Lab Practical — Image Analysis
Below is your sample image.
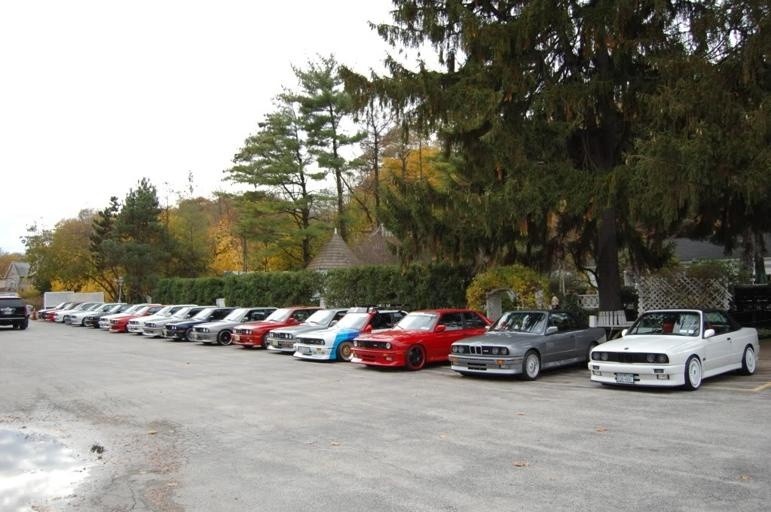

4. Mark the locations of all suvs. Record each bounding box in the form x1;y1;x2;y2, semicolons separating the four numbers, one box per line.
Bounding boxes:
1;291;30;329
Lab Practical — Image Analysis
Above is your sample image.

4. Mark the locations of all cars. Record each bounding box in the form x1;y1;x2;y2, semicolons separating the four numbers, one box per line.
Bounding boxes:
589;308;760;389
449;310;606;380
